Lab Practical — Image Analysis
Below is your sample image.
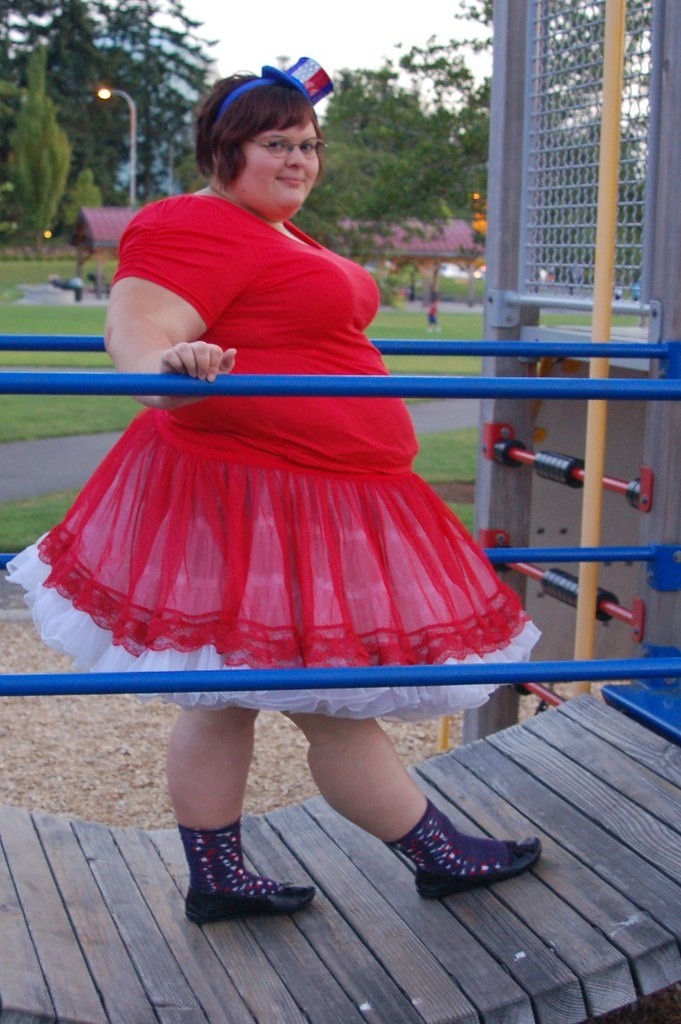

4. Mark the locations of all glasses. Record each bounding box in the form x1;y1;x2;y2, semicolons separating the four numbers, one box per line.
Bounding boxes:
248;137;327;159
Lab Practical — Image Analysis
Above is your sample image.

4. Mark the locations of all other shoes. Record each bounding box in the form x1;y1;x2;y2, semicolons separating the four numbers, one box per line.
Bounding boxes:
186;881;316;923
417;835;542;897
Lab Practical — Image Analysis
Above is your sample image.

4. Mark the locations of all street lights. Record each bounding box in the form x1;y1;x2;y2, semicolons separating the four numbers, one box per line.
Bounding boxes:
97;88;138;207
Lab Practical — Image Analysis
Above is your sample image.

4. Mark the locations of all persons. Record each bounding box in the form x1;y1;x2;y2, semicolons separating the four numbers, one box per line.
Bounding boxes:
5;57;542;923
426;297;440;332
